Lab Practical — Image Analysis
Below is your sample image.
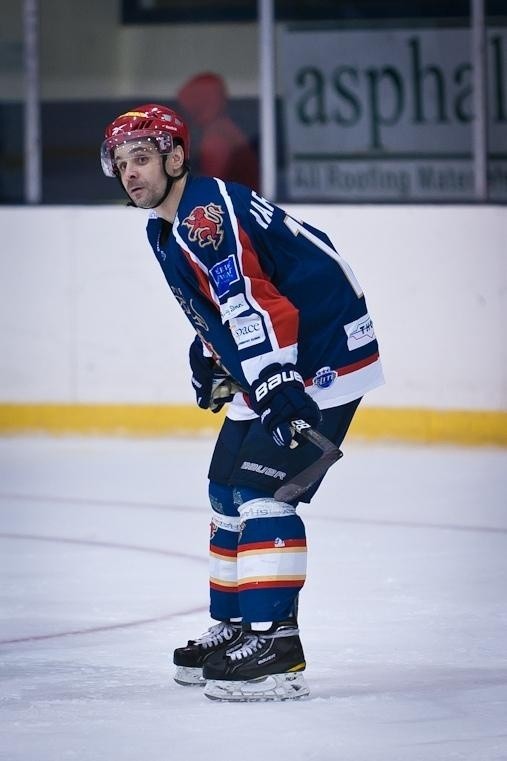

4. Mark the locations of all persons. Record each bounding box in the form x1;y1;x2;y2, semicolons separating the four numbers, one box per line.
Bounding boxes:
175;69;265;196
97;101;389;683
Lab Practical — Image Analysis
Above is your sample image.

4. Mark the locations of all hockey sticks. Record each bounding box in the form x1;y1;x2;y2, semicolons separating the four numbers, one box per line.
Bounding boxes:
225;375;343;501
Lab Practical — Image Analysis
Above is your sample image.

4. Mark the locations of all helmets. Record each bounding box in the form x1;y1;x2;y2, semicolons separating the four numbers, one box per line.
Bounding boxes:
100;103;191;178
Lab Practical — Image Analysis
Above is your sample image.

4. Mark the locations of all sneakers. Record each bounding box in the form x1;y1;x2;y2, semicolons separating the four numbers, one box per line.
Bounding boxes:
173;617;243;668
202;618;307;682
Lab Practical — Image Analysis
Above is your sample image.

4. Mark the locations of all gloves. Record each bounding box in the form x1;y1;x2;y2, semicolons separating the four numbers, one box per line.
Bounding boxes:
189;333;241;414
248;362;323;448
